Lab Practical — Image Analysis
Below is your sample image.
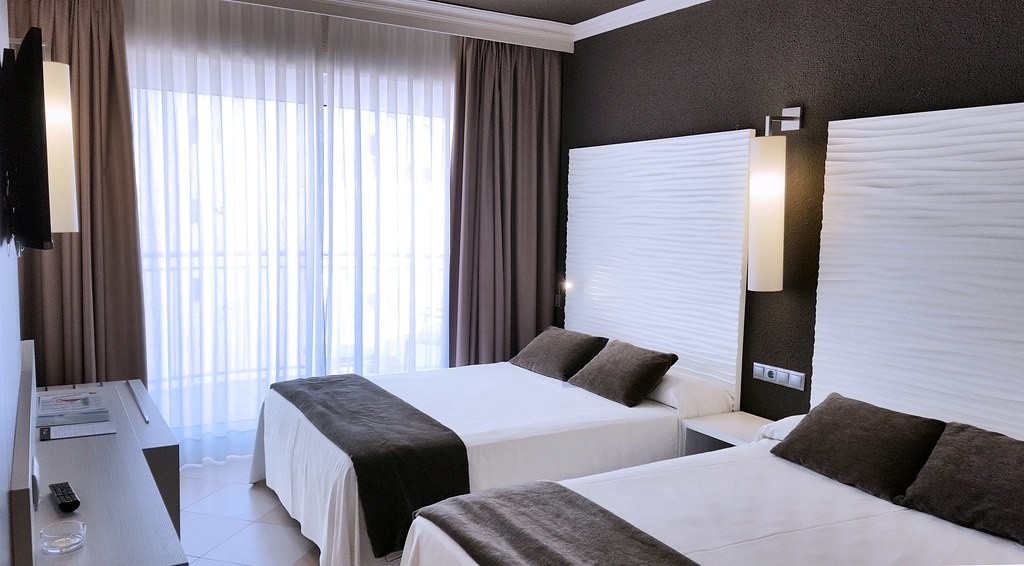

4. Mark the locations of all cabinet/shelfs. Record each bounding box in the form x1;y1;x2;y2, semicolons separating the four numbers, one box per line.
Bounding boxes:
10;339;188;566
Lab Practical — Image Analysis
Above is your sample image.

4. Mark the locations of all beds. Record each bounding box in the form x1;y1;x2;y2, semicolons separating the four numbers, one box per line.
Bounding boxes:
248;361;735;566
398;414;1024;566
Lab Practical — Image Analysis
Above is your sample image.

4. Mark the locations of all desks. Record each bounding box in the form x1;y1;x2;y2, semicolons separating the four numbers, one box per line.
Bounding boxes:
681;410;774;456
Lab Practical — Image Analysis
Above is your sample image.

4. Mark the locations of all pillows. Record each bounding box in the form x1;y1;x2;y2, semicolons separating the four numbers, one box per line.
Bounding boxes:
770;393;948;504
756;414;806;443
893;422;1024;546
650;370;732;418
568;340;679;408
509;326;609;382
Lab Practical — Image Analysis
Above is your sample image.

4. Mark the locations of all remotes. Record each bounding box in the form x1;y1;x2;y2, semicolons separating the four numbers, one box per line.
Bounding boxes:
47;481;82;513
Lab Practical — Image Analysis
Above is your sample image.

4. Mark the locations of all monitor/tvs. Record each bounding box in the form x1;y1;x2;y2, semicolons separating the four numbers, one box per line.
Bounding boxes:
13;28;55;250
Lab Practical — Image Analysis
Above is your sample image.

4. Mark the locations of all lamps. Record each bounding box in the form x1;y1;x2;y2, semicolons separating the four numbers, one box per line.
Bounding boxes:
9;37;79;232
748;107;802;292
